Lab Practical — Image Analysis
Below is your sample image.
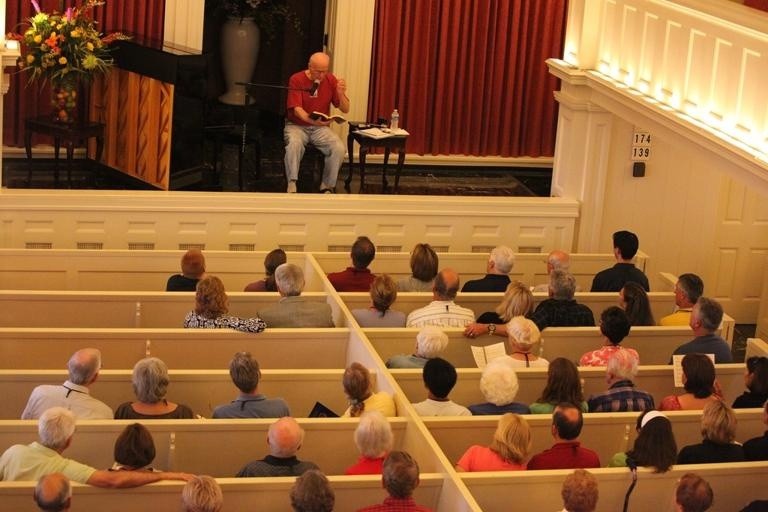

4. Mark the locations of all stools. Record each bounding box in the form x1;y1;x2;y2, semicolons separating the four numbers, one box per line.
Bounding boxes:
268;140;322;191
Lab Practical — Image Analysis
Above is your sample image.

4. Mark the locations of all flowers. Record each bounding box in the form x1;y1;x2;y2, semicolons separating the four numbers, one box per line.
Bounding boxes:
1;1;120;86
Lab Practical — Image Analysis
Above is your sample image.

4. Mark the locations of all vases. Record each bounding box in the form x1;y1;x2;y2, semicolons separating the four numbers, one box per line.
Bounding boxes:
48;83;79;123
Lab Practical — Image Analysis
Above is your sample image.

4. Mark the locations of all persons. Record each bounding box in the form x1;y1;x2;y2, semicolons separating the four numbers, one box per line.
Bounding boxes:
20;347;113;421
0;407;198;490
282;51;350;193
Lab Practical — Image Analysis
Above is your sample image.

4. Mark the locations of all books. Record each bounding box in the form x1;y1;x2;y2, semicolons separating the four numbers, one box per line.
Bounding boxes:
351;127;409;140
309;111;345;124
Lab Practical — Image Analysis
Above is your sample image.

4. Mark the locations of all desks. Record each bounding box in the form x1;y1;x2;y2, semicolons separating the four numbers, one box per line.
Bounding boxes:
18;116;107;185
344;121;406;192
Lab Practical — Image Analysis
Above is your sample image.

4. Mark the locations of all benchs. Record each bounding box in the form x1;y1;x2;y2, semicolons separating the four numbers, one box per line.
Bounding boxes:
0;244;765;510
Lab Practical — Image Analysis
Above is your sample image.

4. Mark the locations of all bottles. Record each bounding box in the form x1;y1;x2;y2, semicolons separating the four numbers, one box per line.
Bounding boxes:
391;108;400;132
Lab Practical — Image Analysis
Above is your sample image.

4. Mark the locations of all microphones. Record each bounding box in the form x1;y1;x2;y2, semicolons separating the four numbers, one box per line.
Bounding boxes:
310;79;320;95
